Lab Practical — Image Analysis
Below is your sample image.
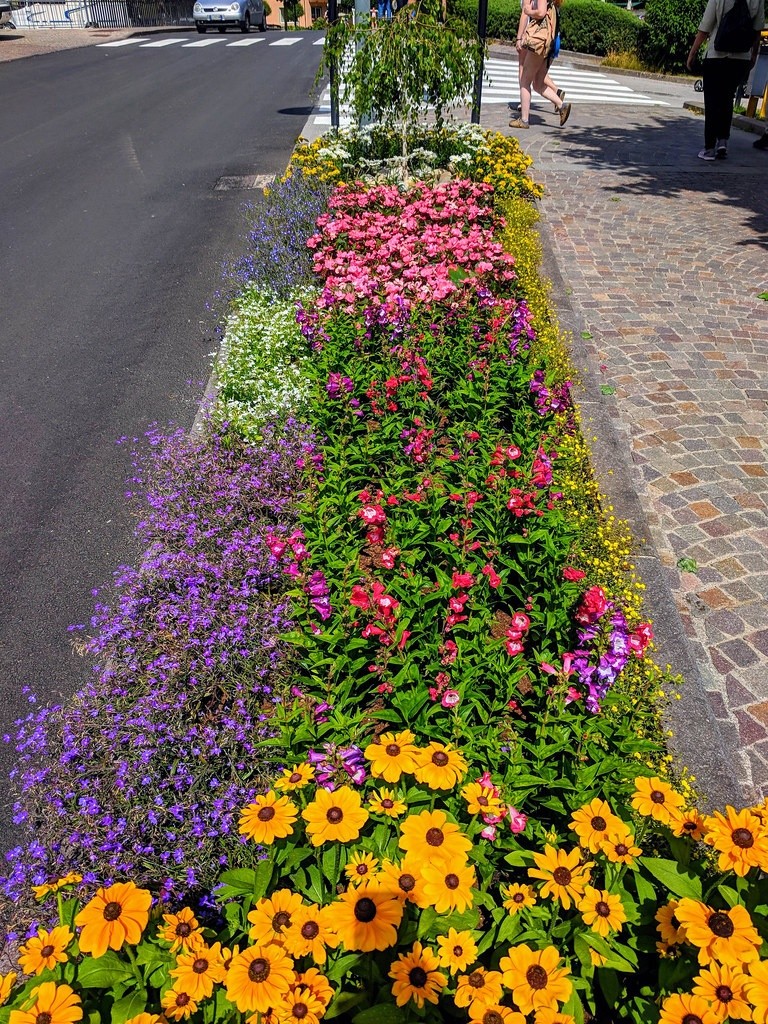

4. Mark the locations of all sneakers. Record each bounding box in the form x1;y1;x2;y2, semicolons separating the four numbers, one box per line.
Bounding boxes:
698;147;716;161
554;89;565;113
716;139;728;160
509;116;530;129
558;103;571;127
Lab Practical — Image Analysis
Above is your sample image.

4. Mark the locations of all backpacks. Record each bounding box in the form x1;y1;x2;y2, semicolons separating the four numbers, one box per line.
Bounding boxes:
714;0;757;54
519;0;556;59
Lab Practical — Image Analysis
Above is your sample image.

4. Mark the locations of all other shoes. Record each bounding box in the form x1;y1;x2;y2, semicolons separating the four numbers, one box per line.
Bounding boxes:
753;130;768;150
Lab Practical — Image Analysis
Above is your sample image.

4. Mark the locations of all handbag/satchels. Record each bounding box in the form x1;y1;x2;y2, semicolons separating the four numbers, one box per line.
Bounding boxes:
549;28;562;59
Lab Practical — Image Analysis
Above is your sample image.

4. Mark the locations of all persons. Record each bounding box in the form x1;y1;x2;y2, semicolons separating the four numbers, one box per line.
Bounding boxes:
686;0;764;160
508;0;565;112
509;0;572;129
370;7;378;29
406;0;447;101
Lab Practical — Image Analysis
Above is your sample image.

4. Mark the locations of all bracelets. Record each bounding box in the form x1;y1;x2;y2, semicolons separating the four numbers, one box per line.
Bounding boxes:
516;38;521;41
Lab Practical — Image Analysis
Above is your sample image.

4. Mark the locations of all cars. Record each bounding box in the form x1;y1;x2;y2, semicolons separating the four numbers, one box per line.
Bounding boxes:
193;0;272;35
